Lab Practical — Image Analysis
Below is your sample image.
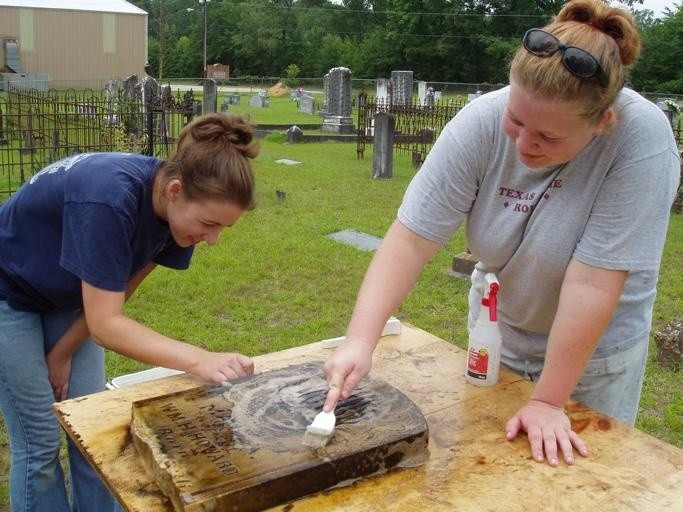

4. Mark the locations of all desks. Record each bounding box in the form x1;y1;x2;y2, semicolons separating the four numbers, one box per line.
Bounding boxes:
51;322;683;511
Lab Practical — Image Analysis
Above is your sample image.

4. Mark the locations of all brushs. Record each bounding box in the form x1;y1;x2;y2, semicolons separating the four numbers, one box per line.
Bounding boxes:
302;399;338;449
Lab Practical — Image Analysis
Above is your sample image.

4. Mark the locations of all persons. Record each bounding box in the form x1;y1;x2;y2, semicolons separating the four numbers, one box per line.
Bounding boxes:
0;111;260;512
323;1;681;467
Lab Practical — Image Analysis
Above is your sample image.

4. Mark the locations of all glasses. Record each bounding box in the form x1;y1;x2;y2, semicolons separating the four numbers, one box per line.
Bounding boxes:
524;28;608;89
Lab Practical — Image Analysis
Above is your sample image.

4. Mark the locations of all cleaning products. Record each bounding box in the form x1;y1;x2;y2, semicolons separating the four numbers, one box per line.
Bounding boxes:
465;272;502;386
